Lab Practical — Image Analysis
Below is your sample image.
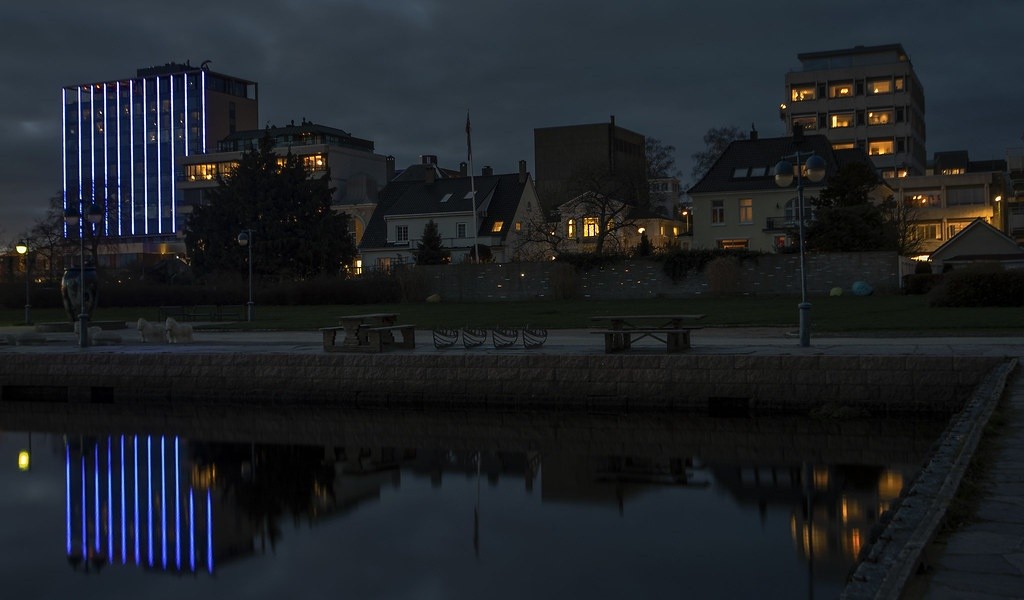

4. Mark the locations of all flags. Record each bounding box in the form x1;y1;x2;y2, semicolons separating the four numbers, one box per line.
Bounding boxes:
465;113;472;163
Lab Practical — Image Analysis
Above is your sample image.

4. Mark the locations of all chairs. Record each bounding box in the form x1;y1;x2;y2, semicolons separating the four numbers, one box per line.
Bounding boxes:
165;317;193;344
137;318;167;344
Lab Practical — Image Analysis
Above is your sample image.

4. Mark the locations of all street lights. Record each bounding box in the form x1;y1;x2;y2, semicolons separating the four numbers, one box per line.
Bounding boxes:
773;150;827;347
238;228;257;321
63;199;103;348
15;238;33;323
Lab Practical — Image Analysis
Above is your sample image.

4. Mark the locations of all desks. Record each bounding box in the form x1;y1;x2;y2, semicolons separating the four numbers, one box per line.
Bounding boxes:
590;313;706;352
340;312;401;352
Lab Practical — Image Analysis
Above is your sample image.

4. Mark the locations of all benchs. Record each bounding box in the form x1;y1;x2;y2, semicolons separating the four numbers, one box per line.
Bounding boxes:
365;324;417;352
87;325;122;345
188;305;218;321
319;324;369;352
432;325;550;350
216;304;244;322
621;326;705;347
590;330;689;352
158;306;188;323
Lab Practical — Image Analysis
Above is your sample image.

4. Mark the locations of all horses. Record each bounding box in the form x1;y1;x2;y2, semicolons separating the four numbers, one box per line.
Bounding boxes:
165;317;195;344
137;317;153;343
